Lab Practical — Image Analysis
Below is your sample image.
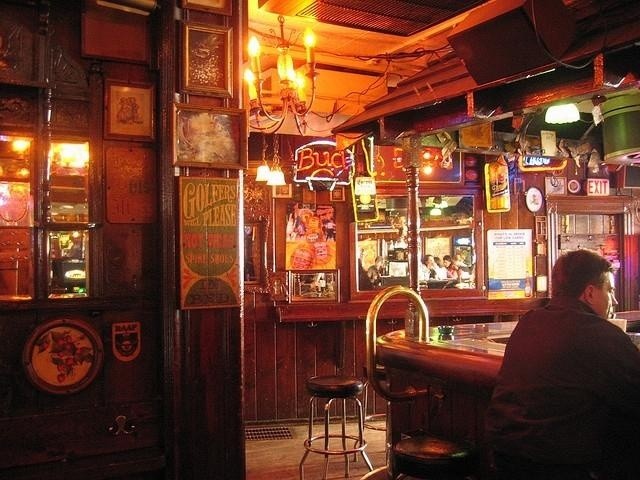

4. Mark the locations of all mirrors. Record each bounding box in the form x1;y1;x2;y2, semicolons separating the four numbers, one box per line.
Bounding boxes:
348;186;487;302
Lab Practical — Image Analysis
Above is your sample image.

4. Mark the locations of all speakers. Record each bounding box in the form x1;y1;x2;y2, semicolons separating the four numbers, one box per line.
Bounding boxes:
446;0;576;85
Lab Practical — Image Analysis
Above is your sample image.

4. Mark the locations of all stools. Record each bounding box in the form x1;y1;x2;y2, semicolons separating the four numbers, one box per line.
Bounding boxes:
391;433;478;480
299;375;374;480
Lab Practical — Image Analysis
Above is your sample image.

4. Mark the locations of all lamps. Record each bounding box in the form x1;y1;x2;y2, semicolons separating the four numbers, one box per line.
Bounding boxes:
245;13;321;188
429;194;450;221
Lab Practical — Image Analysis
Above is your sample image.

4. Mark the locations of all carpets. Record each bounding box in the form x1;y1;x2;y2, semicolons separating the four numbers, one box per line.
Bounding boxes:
243;424;297;443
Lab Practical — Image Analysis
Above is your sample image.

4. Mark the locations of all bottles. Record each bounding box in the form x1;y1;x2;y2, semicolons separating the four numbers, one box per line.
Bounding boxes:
525;271;532;297
348;139;379;224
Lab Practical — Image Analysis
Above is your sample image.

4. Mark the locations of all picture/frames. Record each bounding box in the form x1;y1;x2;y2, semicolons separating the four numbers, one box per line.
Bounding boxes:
171;103;248;170
102;78;157;144
245;216;272;294
287;270;340;304
180;21;233;100
177;176;244;311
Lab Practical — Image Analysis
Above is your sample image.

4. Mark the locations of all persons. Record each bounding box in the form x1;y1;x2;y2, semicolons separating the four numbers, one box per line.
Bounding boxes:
418;254;461;287
367;256;385;288
326;220;336;242
486;248;640;480
